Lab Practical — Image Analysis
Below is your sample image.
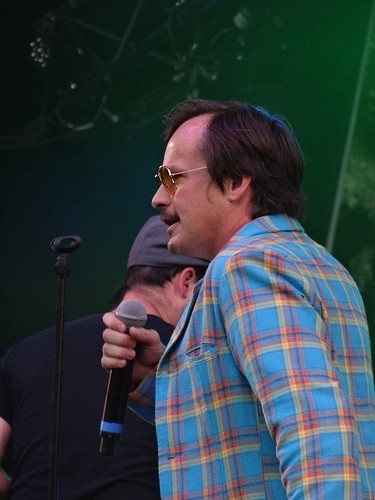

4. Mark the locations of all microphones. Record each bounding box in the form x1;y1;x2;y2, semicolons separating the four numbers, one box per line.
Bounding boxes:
97;300;148;471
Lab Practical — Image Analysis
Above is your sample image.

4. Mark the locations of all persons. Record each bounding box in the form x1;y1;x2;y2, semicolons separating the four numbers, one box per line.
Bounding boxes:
0;214;212;500
100;98;375;500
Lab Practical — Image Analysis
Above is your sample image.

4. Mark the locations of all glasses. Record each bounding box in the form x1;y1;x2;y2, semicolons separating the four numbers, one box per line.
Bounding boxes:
155;165;211;196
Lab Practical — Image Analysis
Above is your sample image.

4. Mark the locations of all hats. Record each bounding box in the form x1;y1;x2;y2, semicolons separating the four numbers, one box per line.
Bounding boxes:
127;215;211;270
